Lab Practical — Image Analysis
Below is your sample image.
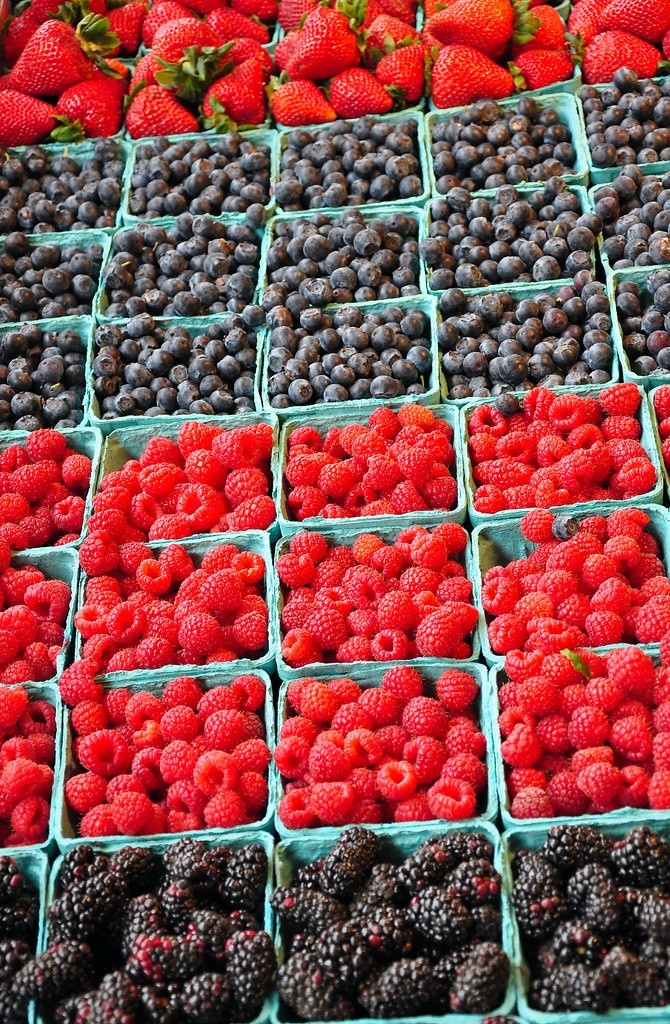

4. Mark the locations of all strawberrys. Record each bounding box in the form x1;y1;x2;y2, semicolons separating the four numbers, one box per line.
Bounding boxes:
0;0;670;150
